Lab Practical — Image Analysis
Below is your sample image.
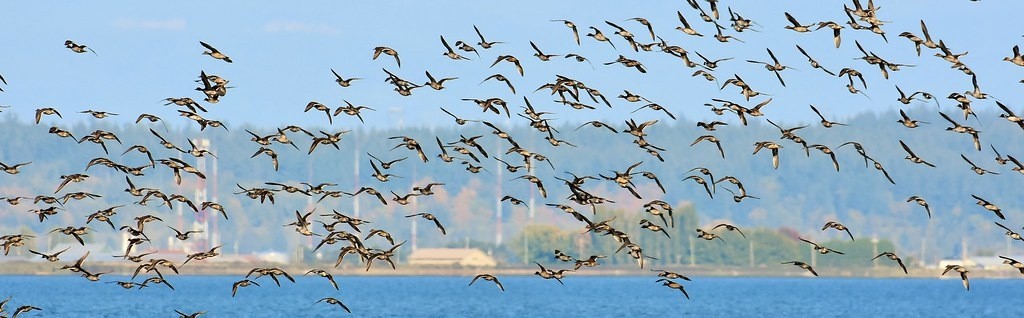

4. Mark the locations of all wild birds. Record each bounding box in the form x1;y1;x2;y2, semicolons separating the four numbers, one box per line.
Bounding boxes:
0;0;1024;318
